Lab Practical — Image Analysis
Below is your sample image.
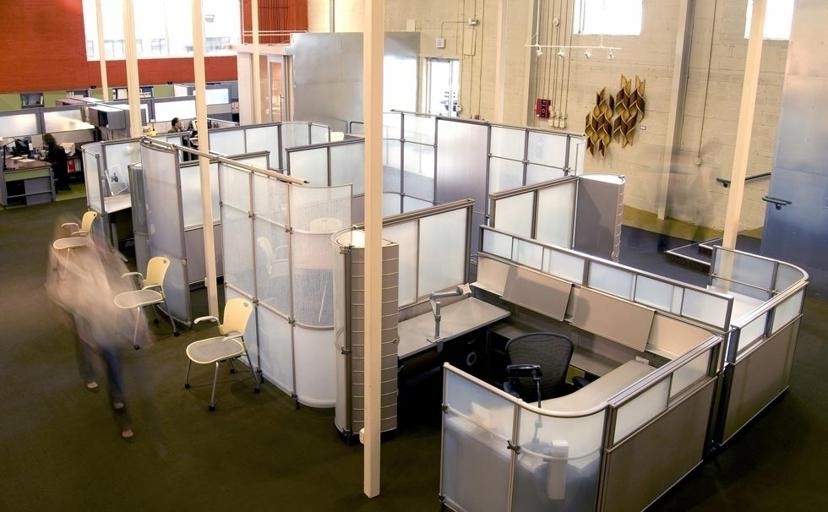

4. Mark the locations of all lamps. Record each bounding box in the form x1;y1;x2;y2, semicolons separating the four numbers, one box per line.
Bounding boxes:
506;364;550;453
522;33;624;62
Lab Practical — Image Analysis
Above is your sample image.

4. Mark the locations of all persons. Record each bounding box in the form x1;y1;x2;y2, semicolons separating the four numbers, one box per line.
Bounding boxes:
45;211;152;444
167;117;185;133
34;133;67;193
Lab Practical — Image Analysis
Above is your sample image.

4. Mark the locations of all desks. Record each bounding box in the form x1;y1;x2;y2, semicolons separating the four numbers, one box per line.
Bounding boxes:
445;359;670;475
6;149;80;208
397;296;512;360
104;192;132;247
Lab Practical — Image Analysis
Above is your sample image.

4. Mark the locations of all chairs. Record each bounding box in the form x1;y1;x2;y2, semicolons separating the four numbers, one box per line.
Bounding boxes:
258;217;342;280
503;330;592;399
184;298;259;408
115;257;179;350
54;211;102;277
